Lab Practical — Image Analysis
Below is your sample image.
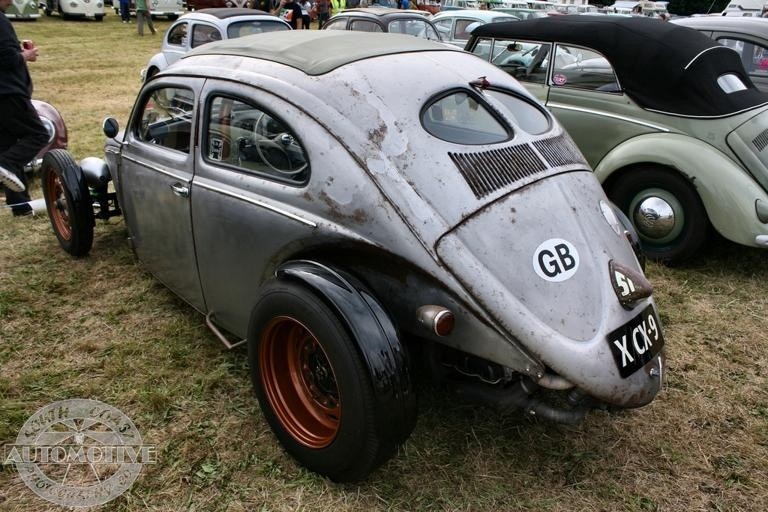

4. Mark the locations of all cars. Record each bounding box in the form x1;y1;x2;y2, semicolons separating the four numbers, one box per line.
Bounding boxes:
2;2;41;22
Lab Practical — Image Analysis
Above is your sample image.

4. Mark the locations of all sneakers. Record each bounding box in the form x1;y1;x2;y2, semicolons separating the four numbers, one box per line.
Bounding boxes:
0;164;26;193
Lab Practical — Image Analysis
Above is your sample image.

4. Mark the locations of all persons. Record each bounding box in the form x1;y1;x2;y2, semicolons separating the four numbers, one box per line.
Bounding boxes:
0;1;53;218
135;1;159;37
120;1;133;24
251;1;428;30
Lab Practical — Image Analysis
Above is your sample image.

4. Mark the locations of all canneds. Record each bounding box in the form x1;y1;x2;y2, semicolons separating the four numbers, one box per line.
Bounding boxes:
23;40;33;50
